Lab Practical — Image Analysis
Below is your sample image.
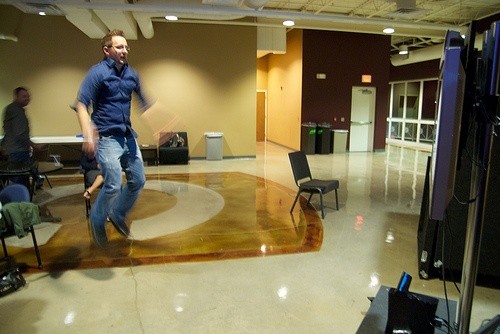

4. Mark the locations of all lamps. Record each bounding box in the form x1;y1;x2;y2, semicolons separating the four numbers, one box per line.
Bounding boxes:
399;44;409;55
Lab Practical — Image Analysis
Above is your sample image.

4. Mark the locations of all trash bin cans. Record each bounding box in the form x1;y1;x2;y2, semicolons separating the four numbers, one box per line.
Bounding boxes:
204;132;223;161
331;129;349;153
318;124;331;153
302;123;317;154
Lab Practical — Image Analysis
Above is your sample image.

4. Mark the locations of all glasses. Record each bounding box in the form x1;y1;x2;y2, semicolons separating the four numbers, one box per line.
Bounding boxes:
107;45;130;52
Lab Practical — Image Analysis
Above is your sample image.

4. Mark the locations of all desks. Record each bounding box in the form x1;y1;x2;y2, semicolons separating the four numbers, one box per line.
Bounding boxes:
356;286;458;334
31;135;85;162
330;130;348;151
140;144;158;166
35;160;64;188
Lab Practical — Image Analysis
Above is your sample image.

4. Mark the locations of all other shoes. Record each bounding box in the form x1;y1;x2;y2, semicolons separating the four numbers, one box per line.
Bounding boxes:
91;221;107;247
107;214;130;237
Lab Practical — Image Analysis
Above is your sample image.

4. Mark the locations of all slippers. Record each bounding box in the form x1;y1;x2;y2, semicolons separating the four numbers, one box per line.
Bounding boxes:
85;191;91;199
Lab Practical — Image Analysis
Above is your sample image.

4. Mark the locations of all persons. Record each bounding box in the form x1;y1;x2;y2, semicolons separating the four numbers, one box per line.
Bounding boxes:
68;28;175;248
0;86;42;236
81;112;123;223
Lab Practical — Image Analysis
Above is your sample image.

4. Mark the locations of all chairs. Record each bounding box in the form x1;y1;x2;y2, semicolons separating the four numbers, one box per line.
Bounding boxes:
0;184;42;269
81;152;123;217
288;151;340;219
159;131;189;164
